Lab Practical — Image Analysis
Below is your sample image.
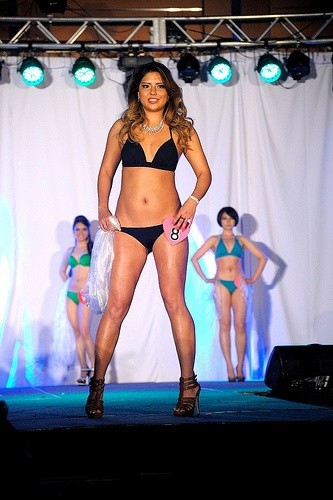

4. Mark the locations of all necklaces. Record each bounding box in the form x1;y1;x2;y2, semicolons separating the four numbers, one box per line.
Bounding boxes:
141;120;164;136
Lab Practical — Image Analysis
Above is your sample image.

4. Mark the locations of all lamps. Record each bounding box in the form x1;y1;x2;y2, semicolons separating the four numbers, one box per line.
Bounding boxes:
168;40;201;83
254;40;287;83
17;43;45;86
118;41;154;92
207;41;232;84
69;44;97;86
283;39;311;81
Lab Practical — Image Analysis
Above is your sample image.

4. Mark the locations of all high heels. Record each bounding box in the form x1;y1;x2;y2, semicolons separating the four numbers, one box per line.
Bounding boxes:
228;365;236;382
88;368;94;384
75;366;91;385
236;366;245;382
174;371;202;416
85;376;105;419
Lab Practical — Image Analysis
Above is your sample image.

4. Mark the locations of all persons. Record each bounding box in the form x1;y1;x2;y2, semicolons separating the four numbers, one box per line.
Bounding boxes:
84;62;211;418
192;207;268;382
59;216;95;383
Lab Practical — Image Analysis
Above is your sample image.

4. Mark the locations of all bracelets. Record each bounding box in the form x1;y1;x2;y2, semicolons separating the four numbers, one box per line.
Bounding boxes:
189;195;199;204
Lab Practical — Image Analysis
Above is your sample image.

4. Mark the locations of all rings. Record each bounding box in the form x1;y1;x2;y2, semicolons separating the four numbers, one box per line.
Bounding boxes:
180;216;184;220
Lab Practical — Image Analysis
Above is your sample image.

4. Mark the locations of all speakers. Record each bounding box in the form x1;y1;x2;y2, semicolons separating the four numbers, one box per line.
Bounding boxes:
264;344;333;395
